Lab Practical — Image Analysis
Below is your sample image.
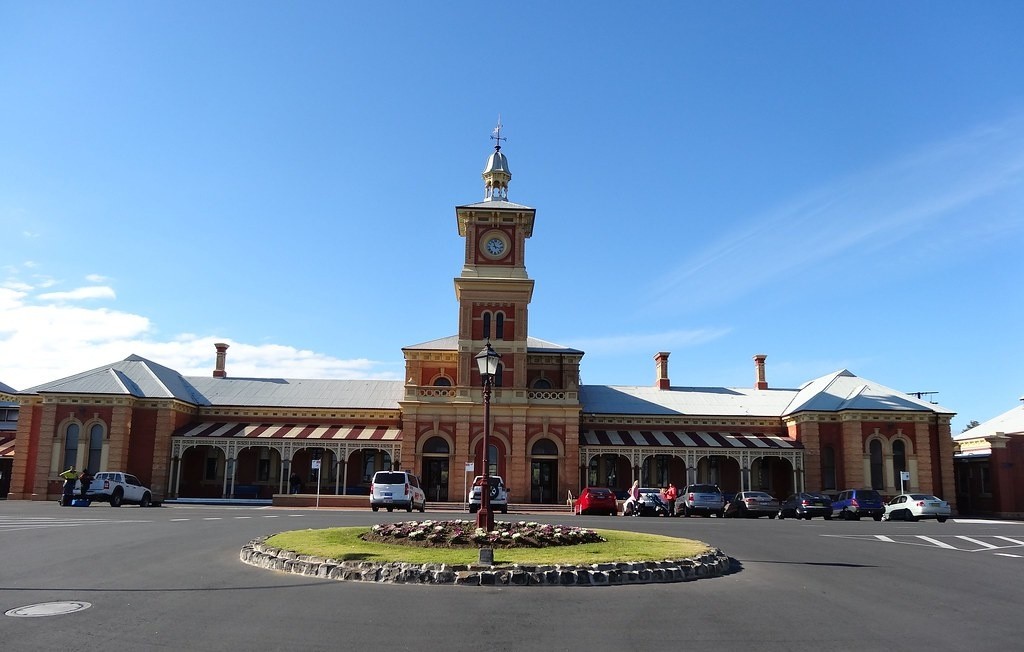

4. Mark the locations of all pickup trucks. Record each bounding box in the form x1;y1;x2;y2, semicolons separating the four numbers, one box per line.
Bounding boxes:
63;472;152;508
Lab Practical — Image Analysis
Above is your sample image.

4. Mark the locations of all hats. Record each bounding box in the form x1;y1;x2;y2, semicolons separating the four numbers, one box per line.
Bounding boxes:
83;468;88;473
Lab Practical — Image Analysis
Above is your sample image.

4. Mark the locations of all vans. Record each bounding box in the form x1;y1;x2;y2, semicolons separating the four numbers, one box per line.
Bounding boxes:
369;471;426;513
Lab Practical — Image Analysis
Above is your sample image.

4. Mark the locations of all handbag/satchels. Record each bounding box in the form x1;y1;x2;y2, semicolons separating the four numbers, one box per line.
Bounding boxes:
71;499;92;507
627;488;642;498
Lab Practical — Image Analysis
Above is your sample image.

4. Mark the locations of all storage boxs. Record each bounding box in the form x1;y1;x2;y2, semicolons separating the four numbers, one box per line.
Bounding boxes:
72;499;89;507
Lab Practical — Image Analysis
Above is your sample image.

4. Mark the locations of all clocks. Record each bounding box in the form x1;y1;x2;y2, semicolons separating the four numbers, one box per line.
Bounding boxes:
487;238;504;255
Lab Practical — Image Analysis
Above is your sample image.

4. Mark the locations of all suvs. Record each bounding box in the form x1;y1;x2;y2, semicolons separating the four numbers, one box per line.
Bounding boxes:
469;476;510;514
673;484;725;518
831;489;886;522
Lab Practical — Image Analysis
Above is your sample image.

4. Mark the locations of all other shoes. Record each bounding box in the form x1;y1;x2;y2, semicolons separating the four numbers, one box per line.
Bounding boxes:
668;513;674;516
632;513;639;516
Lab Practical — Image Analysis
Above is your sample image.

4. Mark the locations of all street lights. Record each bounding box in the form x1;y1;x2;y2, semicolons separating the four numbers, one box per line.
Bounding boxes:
474;337;502;532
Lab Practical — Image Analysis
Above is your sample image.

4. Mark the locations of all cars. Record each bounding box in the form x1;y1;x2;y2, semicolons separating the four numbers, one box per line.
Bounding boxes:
574;487;619;516
778;492;833;520
623;488;668;517
882;493;951;522
733;491;780;519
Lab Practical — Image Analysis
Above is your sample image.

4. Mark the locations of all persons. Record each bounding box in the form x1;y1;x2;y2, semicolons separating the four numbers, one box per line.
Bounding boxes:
665;483;677;517
631;479;642;516
58;465;79;505
80;469;94;501
